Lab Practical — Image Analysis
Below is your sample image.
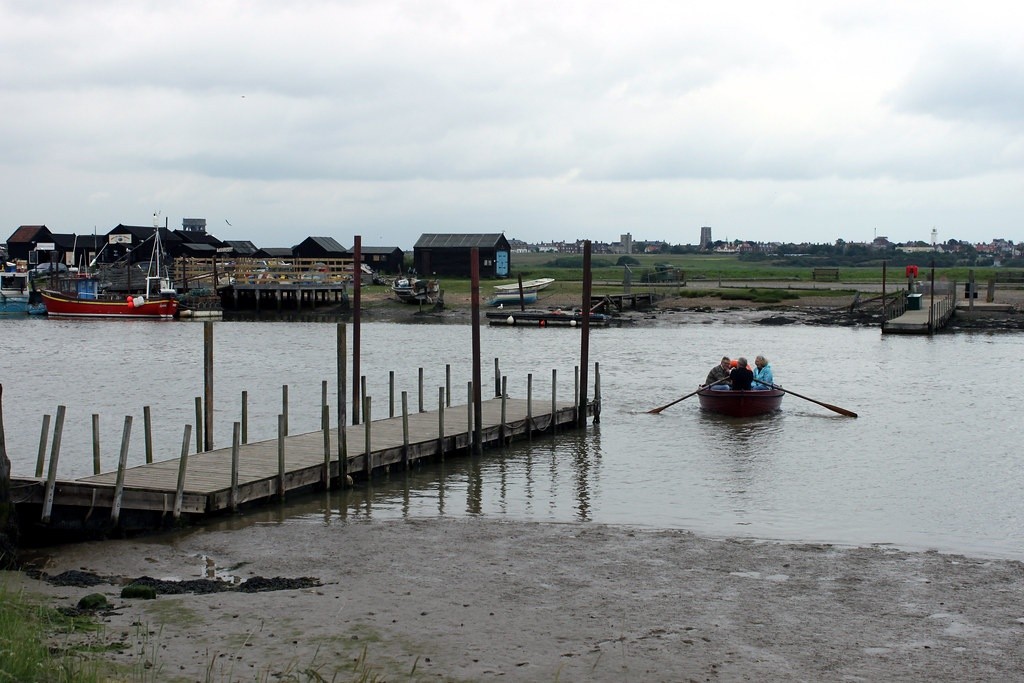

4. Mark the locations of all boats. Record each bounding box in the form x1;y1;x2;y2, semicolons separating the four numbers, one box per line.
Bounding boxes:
390;272;439;303
39;209;180;319
493;277;554;293
185;306;224;317
0;268;30;313
485;287;538;307
697;383;786;417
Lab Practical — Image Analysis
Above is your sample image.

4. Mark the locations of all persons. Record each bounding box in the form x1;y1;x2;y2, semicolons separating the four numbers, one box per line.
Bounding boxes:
730;357;754;390
706;356;732;390
751;356;773;390
907;268;914;290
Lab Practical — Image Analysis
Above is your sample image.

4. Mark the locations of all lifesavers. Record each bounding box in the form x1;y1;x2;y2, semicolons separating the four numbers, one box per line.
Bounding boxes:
76;273;90;276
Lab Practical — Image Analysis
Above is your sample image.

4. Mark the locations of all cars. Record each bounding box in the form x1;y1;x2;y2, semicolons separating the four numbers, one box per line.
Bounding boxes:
28;261;70;279
251;261;269;273
131;260;156;274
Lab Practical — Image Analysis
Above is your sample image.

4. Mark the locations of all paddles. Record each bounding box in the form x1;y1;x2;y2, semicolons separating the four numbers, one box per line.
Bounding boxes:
649;375;732;413
752;377;858;418
